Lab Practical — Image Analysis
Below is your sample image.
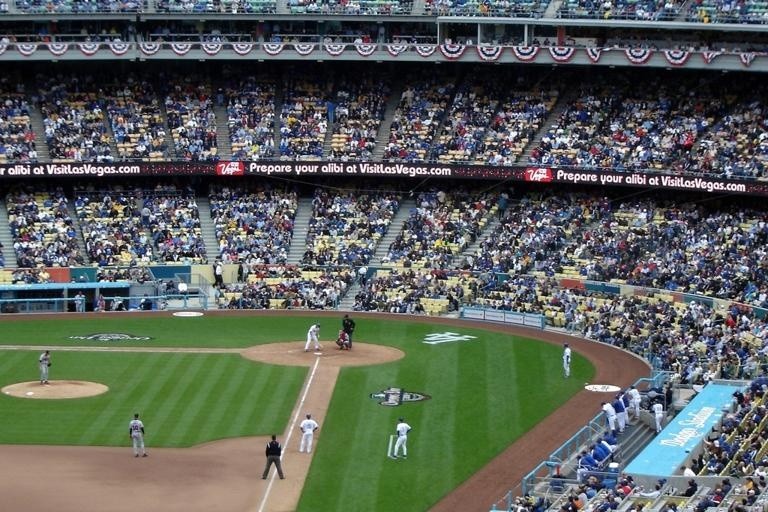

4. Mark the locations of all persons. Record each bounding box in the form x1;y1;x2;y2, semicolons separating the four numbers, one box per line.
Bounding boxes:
304;323;322;352
298;413;319;454
342;313;355;348
37;350;52;385
261;434;285;479
128;413;149;457
336;329;350;350
391;418;412;459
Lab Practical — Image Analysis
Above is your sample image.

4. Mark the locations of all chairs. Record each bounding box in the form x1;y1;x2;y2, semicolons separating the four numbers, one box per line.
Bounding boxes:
229;191;547;315
546;192;767;379
694;389;768;476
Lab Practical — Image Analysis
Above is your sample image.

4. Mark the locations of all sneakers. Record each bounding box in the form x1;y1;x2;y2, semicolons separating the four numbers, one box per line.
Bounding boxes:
136;453;148;456
392;455;408;460
40;380;49;384
